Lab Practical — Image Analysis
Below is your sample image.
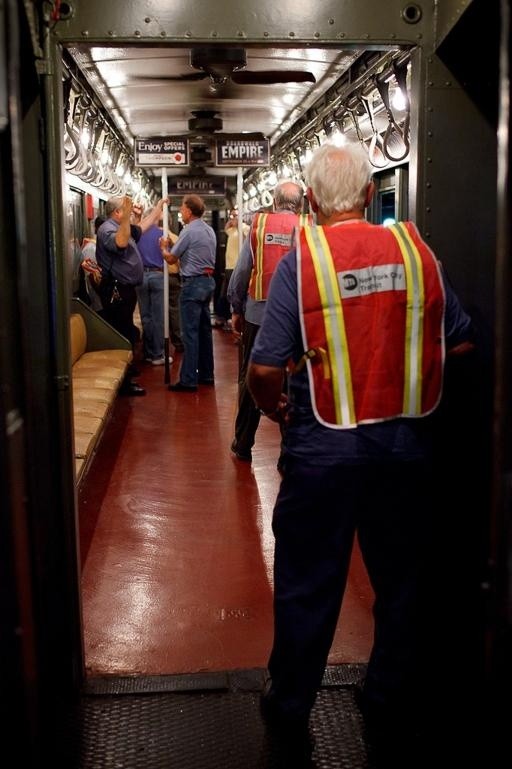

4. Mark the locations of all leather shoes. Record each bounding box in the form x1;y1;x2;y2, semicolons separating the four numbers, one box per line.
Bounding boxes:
127;381;146;399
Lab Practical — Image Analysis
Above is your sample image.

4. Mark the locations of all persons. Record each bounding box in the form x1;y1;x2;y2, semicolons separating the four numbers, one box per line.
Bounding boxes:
226;180;303;464
81;195;248;398
242;142;479;743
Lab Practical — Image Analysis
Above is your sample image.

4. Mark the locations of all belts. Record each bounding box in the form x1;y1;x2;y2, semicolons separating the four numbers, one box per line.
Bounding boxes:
145;268;163;271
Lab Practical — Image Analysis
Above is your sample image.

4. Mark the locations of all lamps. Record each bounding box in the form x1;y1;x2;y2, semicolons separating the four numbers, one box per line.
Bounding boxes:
390;77;406;111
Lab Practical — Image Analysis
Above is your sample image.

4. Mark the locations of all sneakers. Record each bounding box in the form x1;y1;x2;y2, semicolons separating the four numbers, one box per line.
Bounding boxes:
355;678;367;693
261;678;275;697
169;377;214;391
231;439;251;460
145;357;174;365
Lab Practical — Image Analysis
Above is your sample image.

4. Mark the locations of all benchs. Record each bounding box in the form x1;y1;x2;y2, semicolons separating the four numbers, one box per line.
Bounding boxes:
69;313;133;576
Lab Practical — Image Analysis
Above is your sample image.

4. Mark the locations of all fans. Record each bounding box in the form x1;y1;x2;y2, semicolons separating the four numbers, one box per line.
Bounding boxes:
127;46;316;92
147;111;266;142
